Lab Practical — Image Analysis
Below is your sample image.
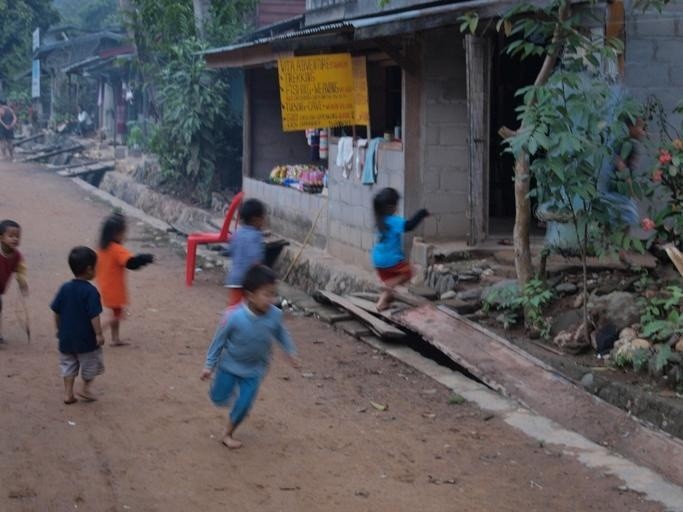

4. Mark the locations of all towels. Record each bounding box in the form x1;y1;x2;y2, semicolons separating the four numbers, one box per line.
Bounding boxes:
335;136;354;180
362;136;384;186
356;138;366;179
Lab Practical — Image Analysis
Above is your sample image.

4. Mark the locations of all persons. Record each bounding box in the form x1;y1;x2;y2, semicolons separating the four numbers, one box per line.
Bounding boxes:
199;264;302;448
0;96;32;161
49;245;104;404
74;104;94;139
223;198;267;307
596;111;647;264
370;187;429;312
93;213;153;346
0;219;30;344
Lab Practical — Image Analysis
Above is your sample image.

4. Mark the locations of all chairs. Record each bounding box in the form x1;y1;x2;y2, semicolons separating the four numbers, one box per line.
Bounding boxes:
185;191;244;287
